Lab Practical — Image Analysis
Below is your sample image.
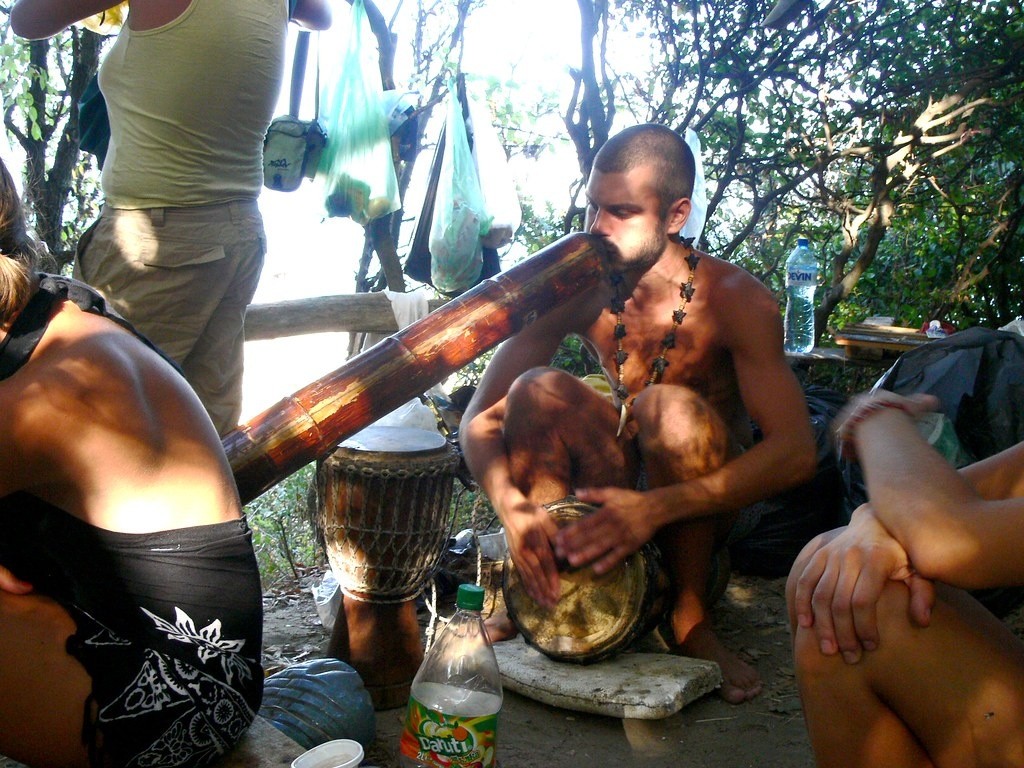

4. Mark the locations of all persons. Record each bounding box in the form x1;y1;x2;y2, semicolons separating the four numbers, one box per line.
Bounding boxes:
12;1;330;445
785;391;1023;768
460;125;815;703
0;158;263;768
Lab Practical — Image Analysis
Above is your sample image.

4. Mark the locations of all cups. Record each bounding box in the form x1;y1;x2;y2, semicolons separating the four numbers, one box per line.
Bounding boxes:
291;739;364;768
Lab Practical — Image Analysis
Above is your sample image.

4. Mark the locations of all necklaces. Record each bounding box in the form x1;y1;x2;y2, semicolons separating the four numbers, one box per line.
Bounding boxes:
608;235;703;439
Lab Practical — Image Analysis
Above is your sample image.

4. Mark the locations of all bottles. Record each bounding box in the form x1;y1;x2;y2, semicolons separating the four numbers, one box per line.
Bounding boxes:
400;584;504;768
782;239;817;354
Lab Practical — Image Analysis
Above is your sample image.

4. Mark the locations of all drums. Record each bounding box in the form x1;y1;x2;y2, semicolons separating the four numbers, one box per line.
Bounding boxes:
490;494;676;663
316;425;459;710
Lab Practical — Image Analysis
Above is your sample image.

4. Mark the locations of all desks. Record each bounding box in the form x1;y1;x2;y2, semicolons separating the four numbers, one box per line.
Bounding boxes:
786;345;894;383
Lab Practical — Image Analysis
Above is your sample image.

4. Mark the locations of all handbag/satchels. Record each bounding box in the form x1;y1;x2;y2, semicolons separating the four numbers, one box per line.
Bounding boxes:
77;69;110;171
262;115;328;192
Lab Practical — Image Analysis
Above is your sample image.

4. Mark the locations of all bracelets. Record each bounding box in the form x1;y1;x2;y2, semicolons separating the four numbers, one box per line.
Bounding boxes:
836;399;916;463
